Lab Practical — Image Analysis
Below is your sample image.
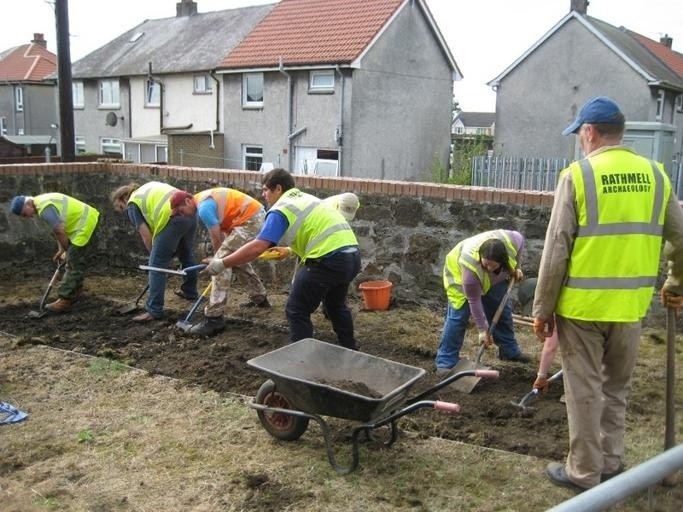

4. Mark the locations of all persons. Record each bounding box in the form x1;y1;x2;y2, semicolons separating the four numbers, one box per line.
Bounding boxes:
526;96;683;497
195;167;362;352
289;193;361;321
108;182;200;323
8;191;102;315
427;227;534;381
515;278;560;398
166;185;274;340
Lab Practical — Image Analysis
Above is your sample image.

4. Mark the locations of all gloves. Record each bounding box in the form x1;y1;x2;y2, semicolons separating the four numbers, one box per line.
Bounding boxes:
532;373;549;402
533;316;555;343
198;257;226;276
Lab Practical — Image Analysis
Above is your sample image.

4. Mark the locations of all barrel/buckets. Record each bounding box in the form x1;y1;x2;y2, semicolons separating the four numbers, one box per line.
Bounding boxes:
358;279;392;312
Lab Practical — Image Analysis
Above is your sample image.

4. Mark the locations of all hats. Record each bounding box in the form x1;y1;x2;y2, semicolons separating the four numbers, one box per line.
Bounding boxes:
169;191;187;217
561;97;625;136
338;193;360;220
11;196;25;216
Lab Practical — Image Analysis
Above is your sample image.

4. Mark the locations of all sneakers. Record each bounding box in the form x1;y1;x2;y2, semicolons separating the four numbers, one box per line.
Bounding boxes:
512;352;529;363
44;298;72;313
239;296;270;309
190;316;226;339
437;368;451;378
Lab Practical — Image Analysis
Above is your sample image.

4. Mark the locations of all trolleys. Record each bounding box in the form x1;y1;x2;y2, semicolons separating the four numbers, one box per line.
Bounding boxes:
244;335;501;476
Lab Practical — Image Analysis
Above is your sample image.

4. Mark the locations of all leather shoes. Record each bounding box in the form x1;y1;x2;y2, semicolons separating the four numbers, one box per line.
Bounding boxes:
132;313;159;322
601;463;624;482
545;461;585;494
175;290;195;300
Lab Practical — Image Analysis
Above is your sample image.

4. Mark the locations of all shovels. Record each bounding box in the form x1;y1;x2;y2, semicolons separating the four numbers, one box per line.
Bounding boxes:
441;271;515;393
174;279;212;331
27;257;66;318
117;282;150;314
138;247;281;276
